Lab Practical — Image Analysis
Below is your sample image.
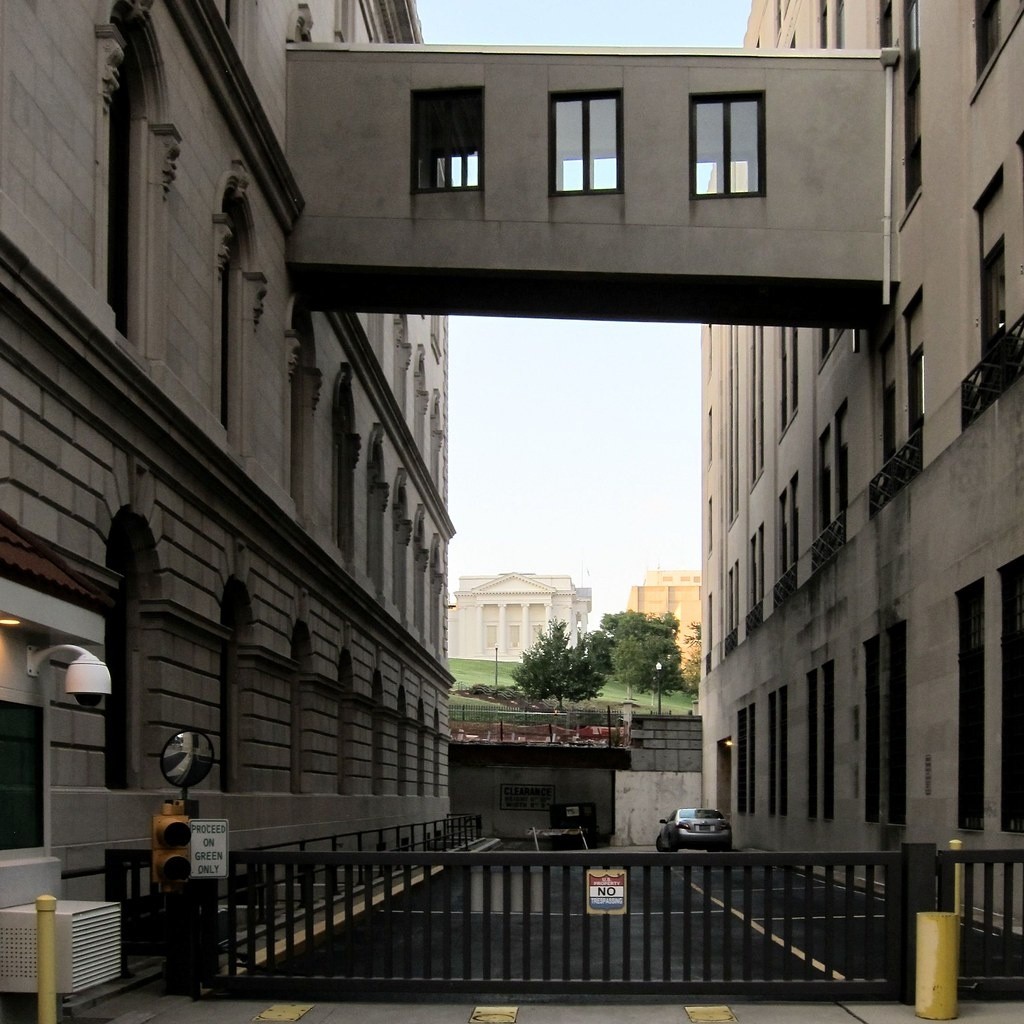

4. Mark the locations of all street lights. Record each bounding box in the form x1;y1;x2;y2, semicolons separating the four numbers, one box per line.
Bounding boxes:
655;662;662;715
494;643;499;685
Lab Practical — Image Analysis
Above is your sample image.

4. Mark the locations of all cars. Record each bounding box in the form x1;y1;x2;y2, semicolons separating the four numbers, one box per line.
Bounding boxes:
655;808;733;852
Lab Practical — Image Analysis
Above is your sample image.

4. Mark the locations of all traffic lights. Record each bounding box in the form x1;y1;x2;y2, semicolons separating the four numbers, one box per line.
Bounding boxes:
150;798;193;894
